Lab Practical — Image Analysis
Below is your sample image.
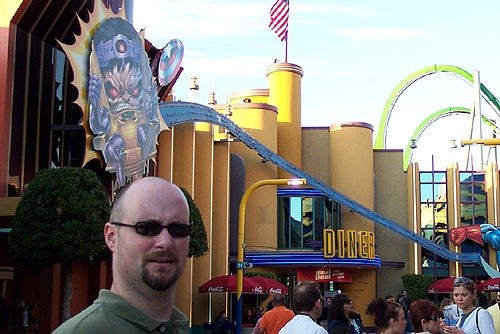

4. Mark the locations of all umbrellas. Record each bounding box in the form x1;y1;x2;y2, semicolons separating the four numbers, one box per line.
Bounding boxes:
429;277;500;293
198;273;288;294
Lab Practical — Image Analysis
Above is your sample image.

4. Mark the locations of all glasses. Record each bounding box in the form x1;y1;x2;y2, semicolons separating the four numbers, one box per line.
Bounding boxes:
454;278;475;291
425;313;441;321
342;299;351;304
110;221;193;237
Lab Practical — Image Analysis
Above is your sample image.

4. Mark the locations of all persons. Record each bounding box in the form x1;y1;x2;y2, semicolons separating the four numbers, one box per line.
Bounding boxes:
410;299;443;334
50;176;193;334
326;290;369;334
442;276;496;334
385;288;500;334
278;280;329;334
365;298;407;334
210;310;228;334
243;293;295;334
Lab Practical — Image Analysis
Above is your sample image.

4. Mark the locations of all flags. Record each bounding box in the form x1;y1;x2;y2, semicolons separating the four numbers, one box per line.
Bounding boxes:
269;0;290;42
